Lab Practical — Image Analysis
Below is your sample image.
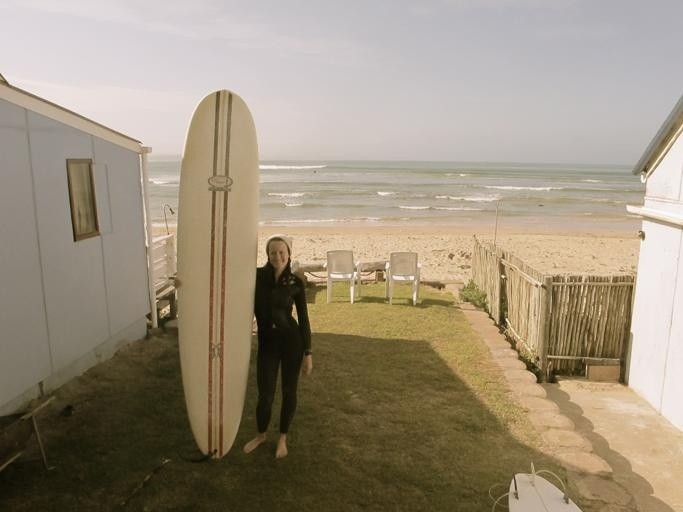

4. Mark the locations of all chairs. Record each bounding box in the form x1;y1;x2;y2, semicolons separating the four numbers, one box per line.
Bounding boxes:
325;250;422;306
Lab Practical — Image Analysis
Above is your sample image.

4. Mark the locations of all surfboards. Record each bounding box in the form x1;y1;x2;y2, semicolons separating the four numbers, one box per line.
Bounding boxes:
177;90;260;460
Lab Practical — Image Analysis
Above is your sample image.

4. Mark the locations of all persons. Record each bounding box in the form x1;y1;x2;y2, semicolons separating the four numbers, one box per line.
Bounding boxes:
174;234;314;458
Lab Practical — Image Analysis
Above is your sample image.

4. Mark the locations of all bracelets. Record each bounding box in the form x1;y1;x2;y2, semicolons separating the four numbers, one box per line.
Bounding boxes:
305;351;313;356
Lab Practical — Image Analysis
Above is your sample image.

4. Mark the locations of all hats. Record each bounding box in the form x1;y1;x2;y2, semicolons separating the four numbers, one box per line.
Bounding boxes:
266;234;293;257
